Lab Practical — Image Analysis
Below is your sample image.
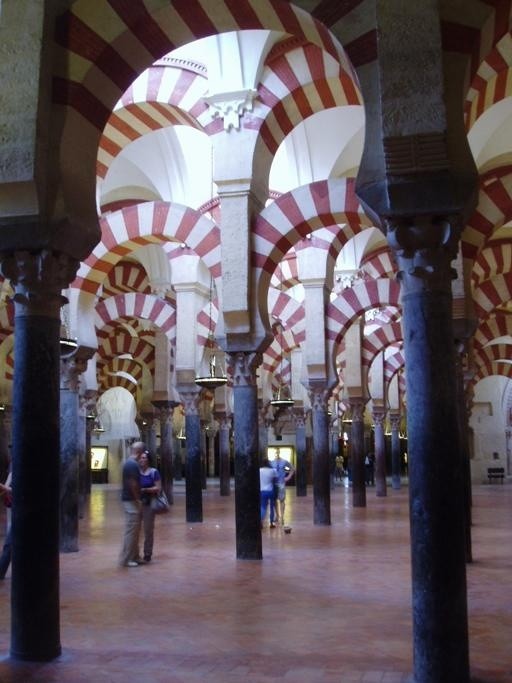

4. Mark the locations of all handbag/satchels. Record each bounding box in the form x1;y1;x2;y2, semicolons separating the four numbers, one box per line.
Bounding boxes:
151;493;170;514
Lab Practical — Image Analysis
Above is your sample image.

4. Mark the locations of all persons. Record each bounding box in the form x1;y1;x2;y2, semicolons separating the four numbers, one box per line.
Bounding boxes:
0;472;12;580
334;451;375;487
490;452;505;488
259;445;295;529
120;442;161;567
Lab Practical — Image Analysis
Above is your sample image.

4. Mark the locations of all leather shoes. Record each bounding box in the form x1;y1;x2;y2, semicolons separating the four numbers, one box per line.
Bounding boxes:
119;560;147;566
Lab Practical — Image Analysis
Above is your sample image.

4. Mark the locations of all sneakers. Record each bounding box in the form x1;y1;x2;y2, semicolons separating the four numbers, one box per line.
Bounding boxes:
144;553;151;560
268;515;284;528
